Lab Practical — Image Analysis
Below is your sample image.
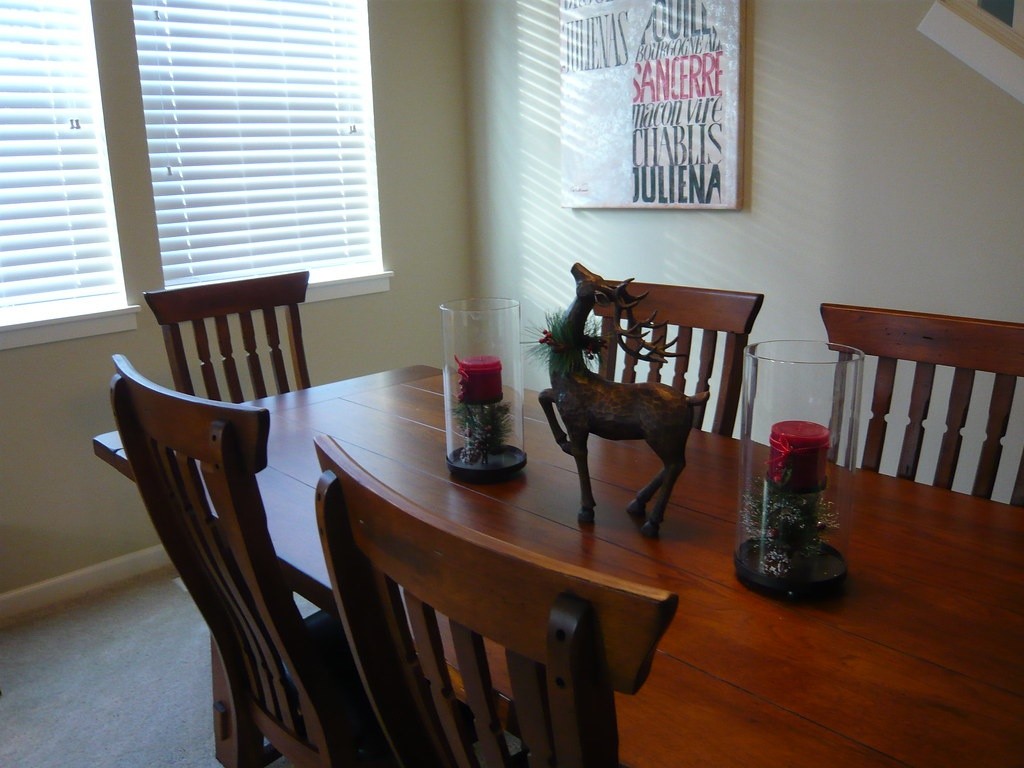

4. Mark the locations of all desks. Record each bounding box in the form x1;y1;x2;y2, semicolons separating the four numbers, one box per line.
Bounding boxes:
87;363;1024;768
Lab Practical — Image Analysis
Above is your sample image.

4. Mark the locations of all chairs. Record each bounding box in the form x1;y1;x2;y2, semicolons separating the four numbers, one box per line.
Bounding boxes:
142;270;310;406
109;353;479;768
593;280;765;437
819;303;1024;506
313;434;679;768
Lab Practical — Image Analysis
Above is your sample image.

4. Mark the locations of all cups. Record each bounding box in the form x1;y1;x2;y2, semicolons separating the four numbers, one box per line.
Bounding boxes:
733;340;868;606
436;297;527;486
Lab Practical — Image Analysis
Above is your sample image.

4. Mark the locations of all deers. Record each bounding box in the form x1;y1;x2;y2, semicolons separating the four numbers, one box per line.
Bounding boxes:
537;262;712;539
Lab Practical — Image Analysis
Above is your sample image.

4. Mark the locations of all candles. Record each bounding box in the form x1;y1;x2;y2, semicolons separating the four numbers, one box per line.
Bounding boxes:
770;421;832;496
458;356;504;407
